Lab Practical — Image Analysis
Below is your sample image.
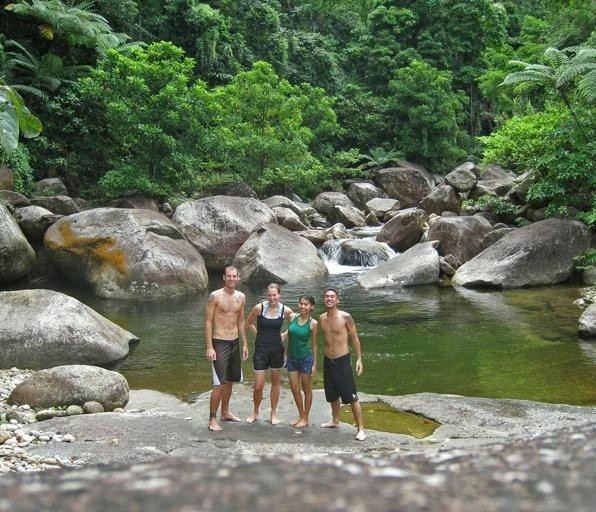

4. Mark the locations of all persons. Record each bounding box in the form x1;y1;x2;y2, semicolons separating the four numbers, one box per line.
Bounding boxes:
317;288;366;440
205;267;249;432
284;296;317;428
246;282;294;425
